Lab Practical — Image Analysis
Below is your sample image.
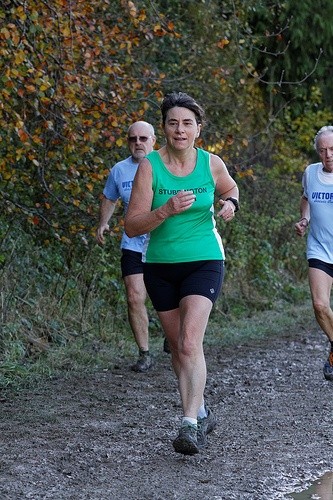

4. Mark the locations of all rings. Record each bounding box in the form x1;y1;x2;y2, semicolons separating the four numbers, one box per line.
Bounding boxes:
227;214;229;218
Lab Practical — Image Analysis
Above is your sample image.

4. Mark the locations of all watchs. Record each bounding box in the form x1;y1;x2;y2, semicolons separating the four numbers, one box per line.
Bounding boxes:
225;197;239;212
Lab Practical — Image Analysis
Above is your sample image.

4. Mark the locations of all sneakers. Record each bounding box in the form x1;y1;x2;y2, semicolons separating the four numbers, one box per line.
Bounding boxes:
197;407;217;441
173;420;199;456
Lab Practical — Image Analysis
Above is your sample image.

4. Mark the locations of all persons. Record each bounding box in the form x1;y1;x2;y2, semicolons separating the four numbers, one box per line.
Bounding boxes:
295;128;333;379
124;94;239;456
98;121;157;370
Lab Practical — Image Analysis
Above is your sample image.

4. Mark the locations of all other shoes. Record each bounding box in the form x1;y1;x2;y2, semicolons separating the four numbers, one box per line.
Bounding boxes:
132;353;153;371
163;338;171;354
323;351;333;379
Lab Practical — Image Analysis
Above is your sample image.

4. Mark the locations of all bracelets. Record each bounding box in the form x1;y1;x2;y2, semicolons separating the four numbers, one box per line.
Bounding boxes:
300;218;309;226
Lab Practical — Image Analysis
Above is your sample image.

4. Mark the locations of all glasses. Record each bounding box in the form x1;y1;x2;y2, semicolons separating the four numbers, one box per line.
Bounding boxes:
128;136;152;142
